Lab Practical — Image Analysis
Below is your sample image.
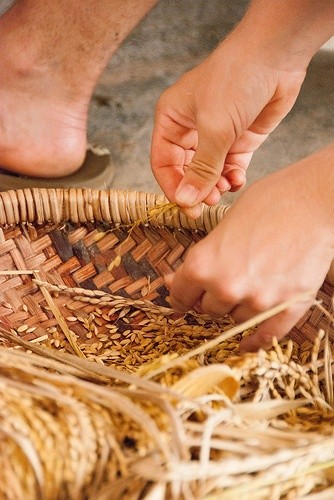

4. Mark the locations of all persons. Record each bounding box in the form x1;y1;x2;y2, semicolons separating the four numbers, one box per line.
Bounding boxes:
1;0;334;352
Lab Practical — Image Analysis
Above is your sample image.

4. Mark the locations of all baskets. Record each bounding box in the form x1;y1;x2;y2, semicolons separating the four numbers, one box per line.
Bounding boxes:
1;189;332;401
